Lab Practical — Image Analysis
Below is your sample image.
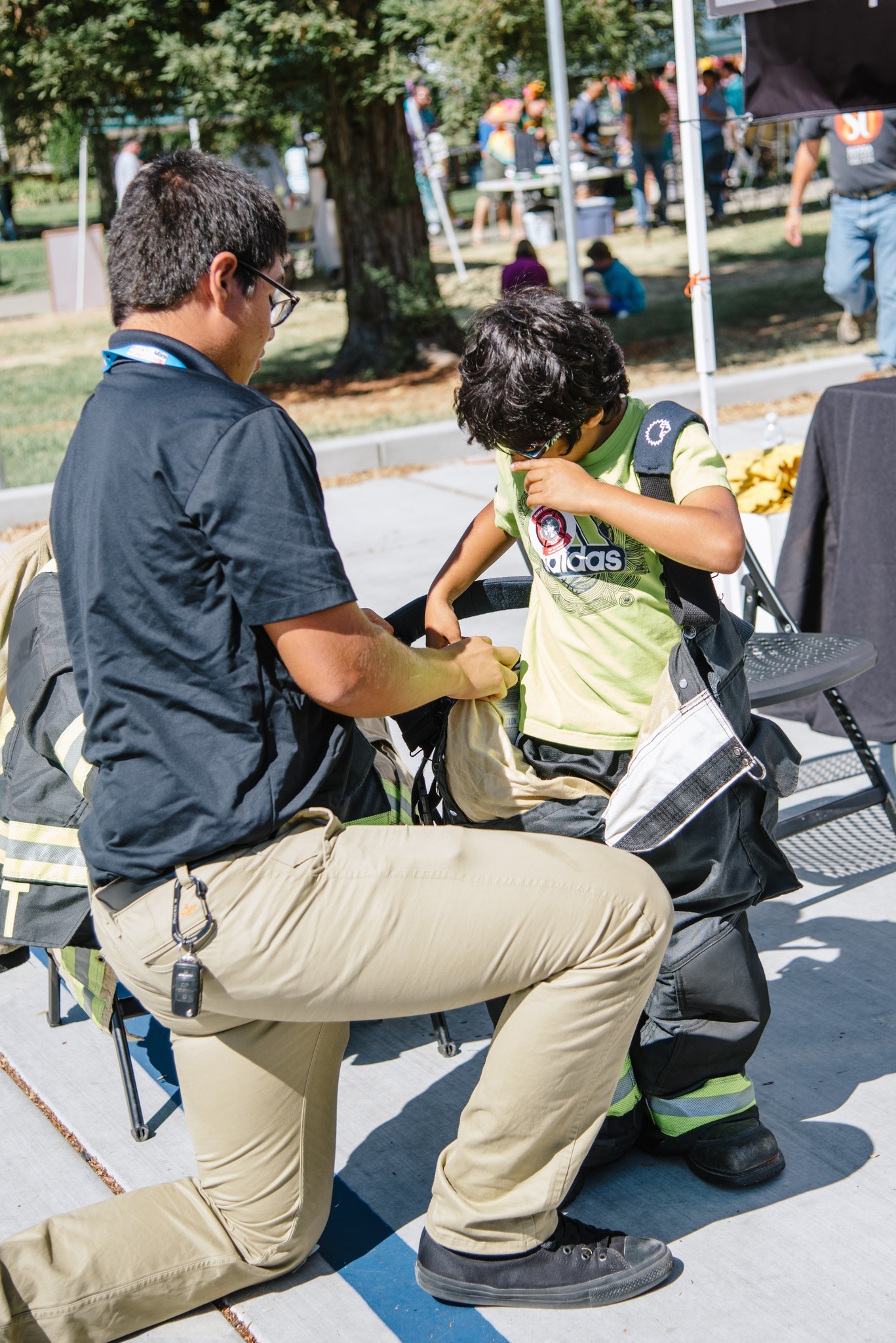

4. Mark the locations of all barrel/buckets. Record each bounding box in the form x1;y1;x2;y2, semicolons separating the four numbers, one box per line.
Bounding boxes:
578;196;616;238
524;207;556;246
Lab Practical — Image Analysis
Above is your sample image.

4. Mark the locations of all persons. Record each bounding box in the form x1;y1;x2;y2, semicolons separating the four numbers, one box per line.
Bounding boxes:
112;135;142;209
578;237;649;323
786;107;895;378
501;237;545;302
0;148;685;1343
425;294;800;1194
387;52;767;229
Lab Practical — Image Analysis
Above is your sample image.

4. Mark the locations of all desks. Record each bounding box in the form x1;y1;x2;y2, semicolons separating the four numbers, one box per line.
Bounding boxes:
476;164;615;244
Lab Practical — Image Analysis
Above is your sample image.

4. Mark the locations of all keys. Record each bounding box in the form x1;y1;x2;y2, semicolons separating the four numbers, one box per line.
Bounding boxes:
169;958;205;1023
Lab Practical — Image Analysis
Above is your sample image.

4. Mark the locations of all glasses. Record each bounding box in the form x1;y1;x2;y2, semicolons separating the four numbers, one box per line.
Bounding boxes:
494;424;561;460
237;260;301;328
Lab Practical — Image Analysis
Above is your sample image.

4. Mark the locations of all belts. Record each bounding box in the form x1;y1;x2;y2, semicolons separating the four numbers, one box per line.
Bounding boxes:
834;181;896;200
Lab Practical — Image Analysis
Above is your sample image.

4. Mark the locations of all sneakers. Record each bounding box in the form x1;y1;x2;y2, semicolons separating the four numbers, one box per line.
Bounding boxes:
556;1104;647;1213
878;362;896;380
651;1108;786;1189
414;1212;675;1309
837;299;877;345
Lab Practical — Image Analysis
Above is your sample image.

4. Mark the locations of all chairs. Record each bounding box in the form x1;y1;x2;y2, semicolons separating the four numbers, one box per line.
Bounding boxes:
704;532;896;850
